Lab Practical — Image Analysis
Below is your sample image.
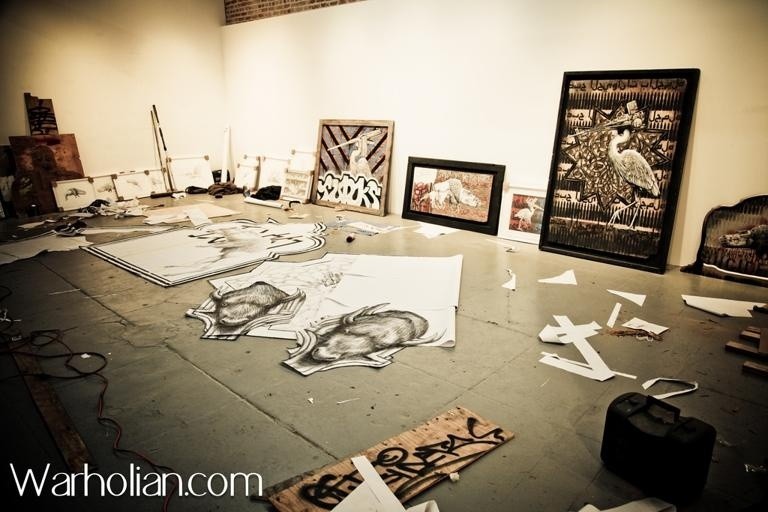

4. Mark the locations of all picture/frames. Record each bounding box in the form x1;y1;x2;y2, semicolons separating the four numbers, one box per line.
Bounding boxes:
51;149;316;213
680;195;768;286
311;119;394;216
498;186;547;244
539;68;700;274
402;156;505;236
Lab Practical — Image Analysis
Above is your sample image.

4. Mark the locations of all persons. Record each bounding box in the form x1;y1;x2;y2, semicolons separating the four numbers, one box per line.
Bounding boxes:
348;134;372;177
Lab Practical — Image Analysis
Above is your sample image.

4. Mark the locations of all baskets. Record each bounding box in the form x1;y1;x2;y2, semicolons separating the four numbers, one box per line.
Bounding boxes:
599;386;719;505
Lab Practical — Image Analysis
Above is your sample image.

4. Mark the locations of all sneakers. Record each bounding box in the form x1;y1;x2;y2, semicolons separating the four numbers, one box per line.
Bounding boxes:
52;220;92;237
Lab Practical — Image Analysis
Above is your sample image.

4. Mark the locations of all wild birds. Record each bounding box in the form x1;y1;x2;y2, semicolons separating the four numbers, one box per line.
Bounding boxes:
327;130;381;180
514;205;544;230
587;126;661;229
